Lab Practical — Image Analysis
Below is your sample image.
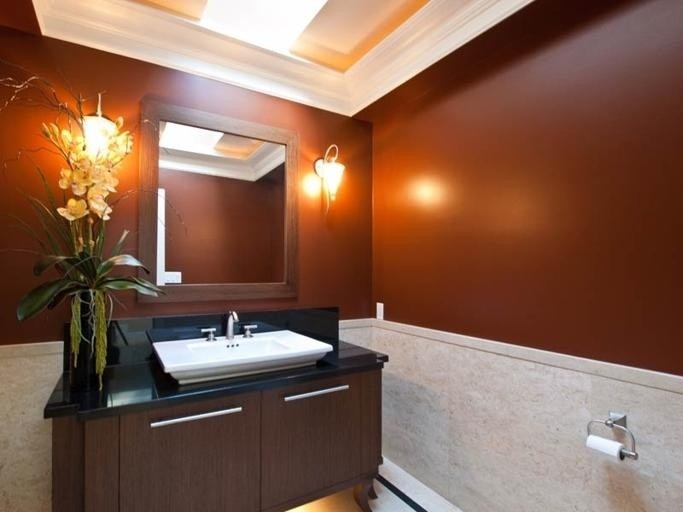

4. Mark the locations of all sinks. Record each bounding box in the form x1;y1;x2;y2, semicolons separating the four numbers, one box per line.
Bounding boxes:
151;328;333;386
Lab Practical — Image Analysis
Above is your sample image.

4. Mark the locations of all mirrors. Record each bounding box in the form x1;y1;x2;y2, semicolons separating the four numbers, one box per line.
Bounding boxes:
137;98;298;304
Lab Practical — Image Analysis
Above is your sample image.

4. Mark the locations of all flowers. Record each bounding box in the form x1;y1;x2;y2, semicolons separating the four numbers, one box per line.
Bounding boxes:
0;67;166;390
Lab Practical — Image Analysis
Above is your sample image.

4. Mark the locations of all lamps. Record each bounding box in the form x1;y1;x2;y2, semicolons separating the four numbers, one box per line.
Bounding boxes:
313;145;347;213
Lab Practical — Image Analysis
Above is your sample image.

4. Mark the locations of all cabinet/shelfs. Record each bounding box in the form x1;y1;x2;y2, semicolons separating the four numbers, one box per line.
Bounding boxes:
51;367;380;507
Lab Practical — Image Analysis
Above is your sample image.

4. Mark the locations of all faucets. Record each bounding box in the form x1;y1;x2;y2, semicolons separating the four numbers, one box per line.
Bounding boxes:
225;310;239;339
